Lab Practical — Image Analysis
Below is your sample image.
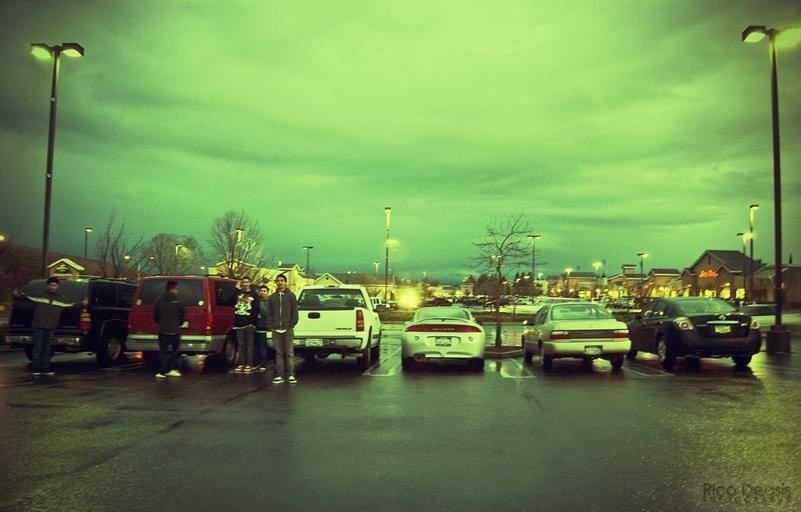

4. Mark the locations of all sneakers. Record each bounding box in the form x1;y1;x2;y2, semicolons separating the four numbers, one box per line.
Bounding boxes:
233;364;297;384
31;369;56;376
152;367;182;379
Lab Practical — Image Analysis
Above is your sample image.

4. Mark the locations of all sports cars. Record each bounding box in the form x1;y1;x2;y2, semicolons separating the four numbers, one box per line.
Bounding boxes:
401;306;489;371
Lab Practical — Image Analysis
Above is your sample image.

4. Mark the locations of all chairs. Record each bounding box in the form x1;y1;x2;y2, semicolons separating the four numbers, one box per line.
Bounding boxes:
345;299;358;307
303;294;319;305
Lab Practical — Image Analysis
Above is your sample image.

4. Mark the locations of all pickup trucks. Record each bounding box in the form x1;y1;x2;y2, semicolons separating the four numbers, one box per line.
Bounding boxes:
264;284;383;367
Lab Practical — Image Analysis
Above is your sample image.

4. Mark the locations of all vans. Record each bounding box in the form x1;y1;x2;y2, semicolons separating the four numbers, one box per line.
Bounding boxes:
345;297;383;310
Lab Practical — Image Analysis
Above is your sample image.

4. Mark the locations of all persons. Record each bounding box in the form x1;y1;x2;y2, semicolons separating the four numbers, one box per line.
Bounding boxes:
224;277;259;374
11;276;88;376
267;275;298;384
254;285;269;370
151;281;187;378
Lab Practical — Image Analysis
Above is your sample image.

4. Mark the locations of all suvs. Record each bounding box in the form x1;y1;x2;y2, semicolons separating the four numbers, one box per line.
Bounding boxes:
123;272;241;366
5;275;137;364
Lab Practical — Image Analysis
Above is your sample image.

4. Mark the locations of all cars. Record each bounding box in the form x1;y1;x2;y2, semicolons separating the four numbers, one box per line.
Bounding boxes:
423;295;651;308
625;296;764;369
520;301;634;373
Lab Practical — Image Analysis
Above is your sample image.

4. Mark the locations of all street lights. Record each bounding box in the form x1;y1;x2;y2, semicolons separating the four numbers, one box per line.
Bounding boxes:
637;251;648;307
30;38;86;282
302;245;315;278
234;228;249;272
491;254;502;267
741;21;801;354
592;260;604;288
502;272;543;294
527;233;543;285
372;261;382;275
564;265;574;291
380;206;395;313
124;255;155;282
83;227;95;273
745;200;762;294
736;231;750;297
175;243;181;266
